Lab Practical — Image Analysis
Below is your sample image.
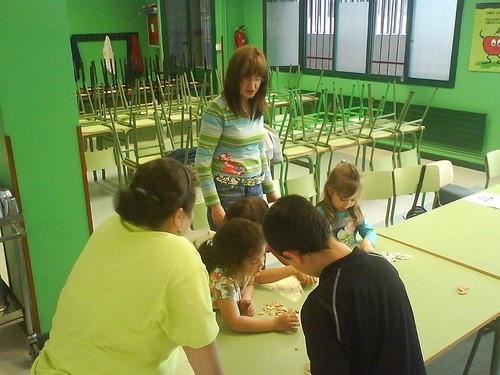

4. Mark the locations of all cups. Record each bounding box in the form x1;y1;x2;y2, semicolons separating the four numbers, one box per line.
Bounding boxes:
3;197;21;217
0;189;13;205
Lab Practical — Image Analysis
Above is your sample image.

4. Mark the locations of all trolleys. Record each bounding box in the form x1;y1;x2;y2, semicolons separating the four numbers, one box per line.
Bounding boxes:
0;190;41;364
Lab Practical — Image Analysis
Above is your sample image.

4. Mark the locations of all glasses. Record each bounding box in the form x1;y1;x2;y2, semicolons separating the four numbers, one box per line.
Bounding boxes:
241;77;265;83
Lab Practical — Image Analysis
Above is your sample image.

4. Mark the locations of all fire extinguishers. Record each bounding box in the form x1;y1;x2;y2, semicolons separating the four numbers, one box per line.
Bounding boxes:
234;24;247;47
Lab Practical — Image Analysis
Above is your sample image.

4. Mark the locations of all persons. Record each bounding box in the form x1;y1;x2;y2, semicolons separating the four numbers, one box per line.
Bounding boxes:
262;194;426;375
316;159;377;254
195;44;279;269
197;218;301;333
30;157;224;375
224;196;316;288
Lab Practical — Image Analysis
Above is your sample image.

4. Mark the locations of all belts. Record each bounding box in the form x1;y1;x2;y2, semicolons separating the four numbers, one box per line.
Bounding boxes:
214;171;266;186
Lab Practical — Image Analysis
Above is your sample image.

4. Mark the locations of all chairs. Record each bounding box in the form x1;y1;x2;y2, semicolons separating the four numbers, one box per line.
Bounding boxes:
74;53;500;274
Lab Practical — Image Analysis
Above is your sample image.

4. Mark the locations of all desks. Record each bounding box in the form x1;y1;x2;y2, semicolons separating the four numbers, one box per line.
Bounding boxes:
158;234;500;375
76;78;426;207
373;184;500;279
